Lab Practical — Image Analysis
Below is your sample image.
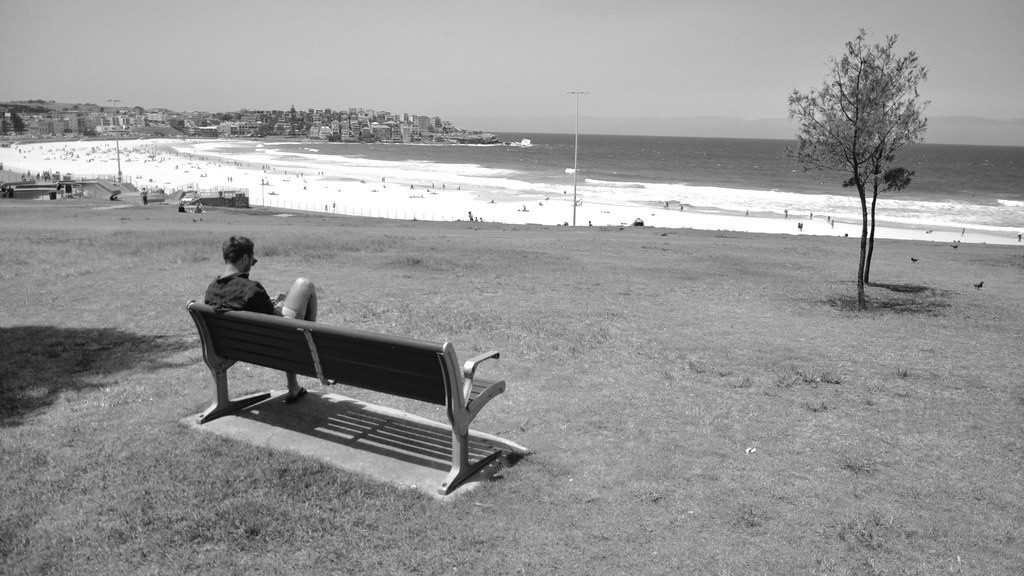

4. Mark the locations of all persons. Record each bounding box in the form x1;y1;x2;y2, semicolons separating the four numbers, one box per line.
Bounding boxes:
1;168;203;214
203;234;318;402
469;211;483;222
744;206;1022;244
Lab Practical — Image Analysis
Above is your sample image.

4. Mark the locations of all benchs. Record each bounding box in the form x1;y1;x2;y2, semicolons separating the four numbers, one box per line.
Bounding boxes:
184;300;506;494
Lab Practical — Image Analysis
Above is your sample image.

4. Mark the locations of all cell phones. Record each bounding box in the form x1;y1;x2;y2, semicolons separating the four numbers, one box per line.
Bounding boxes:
274;294;287;307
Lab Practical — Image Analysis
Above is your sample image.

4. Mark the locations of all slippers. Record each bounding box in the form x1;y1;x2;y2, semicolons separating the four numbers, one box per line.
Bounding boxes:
284;387;307;401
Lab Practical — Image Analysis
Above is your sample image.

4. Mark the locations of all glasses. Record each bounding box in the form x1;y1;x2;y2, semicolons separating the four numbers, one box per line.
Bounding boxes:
242;253;257;266
